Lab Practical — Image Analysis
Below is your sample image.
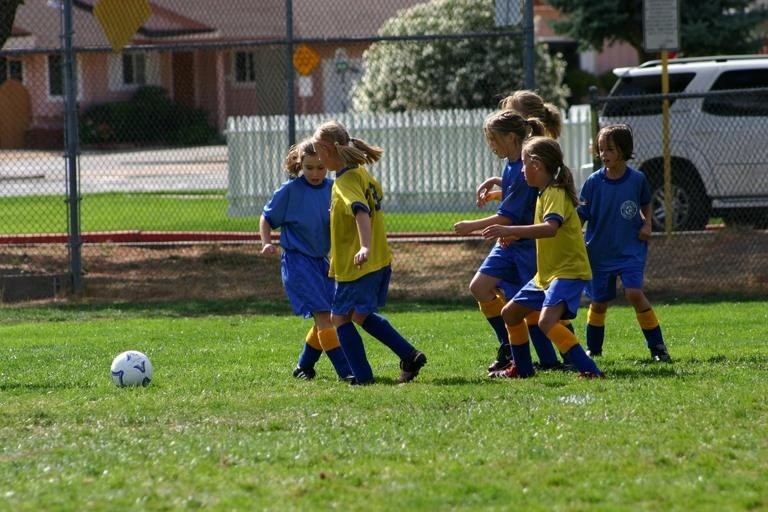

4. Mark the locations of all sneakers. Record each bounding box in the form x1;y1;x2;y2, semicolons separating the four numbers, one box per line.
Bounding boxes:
577;372;605;379
292;366;315;380
556;363;577;371
585;349;602;356
398;351;427;383
488;356;513;370
337;374;356;383
531;362;561;371
651;349;670;362
487;364;519;378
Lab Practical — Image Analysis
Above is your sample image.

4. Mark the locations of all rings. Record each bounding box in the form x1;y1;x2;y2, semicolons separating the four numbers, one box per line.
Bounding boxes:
495;231;498;235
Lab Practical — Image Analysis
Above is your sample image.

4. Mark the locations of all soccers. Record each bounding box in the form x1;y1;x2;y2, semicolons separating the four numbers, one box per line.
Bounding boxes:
111;350;152;388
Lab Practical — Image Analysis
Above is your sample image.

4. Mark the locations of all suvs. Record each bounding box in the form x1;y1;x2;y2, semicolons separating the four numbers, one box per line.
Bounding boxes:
595;52;768;230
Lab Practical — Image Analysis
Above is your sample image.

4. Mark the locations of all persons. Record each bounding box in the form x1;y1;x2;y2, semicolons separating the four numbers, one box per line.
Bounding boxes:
576;123;672;362
453;110;559;370
311;121;425;387
480;137;604;380
259;136;355;382
476;89;574;357
